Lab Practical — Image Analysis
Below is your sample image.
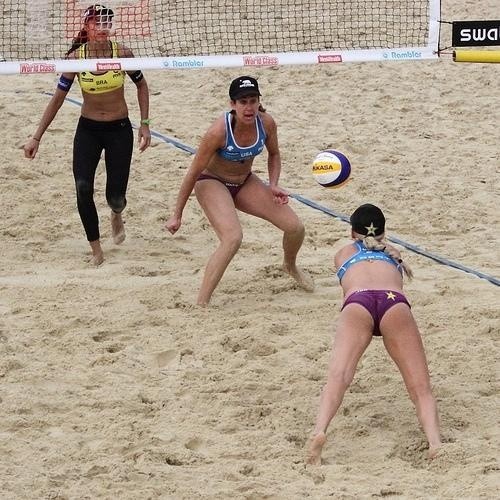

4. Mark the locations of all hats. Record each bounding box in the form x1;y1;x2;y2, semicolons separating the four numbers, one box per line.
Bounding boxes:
84;5;114;23
230;76;262;100
350;204;385;236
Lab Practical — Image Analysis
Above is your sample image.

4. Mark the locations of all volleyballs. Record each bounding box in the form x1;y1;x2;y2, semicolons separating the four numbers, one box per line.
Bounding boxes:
311;149;351;186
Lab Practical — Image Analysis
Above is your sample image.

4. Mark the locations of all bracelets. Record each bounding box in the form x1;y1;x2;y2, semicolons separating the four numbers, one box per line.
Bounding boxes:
33;137;41;142
140;118;151;125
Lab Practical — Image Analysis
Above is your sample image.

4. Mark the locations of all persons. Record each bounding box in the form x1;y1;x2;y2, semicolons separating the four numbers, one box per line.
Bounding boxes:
163;75;312;306
303;203;443;467
22;4;152;266
